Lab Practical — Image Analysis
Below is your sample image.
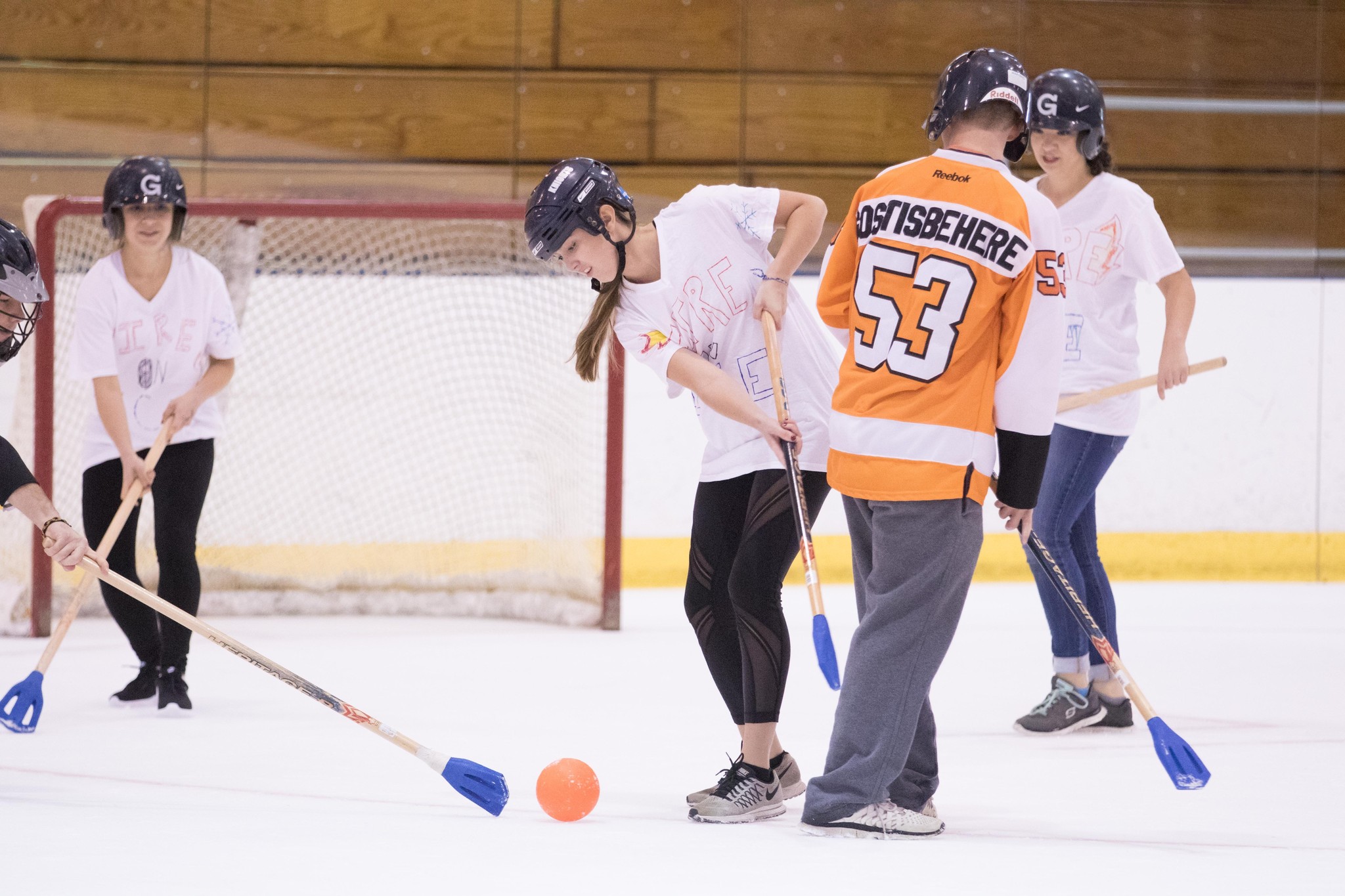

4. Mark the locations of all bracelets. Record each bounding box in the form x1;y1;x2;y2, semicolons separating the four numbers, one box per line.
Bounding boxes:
41;517;72;538
751;268;789;287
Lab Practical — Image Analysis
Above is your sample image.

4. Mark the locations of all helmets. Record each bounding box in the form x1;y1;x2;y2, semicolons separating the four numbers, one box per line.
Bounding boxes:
926;48;1033;163
525;158;634;261
1030;68;1106;158
0;219;49;363
102;154;188;243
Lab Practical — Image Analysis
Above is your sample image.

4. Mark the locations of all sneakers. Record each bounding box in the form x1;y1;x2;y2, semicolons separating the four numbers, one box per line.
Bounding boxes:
156;666;193;716
1015;676;1108;732
799;797;947;842
1089;696;1133;728
688;751;805;807
113;663;156;704
688;752;788;823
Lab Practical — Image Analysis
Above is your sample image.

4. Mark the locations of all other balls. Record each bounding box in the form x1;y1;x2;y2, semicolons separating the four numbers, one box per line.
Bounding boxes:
536;758;600;823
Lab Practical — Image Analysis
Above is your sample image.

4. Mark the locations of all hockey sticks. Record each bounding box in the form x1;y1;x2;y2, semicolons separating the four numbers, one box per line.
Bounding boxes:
761;305;844;692
988;470;1214;790
0;415;179;734
1054;354;1232;415
41;535;509;817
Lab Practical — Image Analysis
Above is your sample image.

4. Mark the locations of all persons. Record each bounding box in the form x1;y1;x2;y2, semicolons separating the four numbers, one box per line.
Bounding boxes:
76;152;235;719
525;155;832;826
1013;69;1195;741
797;32;1067;839
0;217;109;577
